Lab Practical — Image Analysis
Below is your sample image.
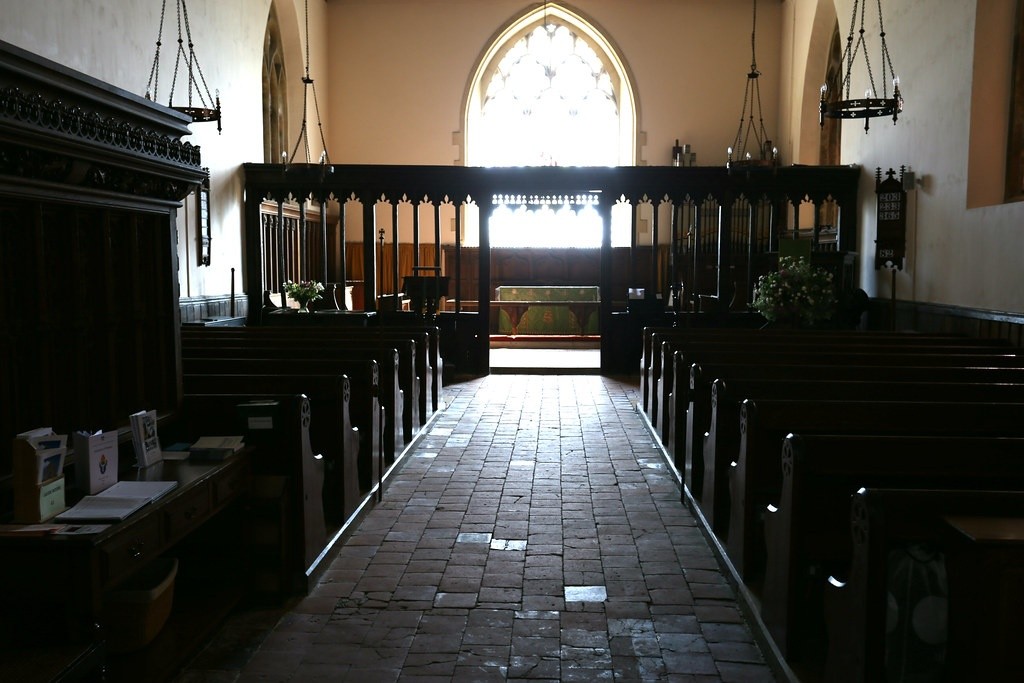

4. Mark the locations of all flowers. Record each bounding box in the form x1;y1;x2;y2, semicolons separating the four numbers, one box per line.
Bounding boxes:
283;280;326;303
747;254;841;323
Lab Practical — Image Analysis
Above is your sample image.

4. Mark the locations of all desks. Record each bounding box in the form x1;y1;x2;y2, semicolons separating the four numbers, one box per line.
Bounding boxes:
0;444;256;683
938;512;1024;683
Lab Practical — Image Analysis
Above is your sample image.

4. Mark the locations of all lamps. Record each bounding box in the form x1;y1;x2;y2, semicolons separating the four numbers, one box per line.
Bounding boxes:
726;0;778;174
141;0;222;132
278;0;334;180
819;0;903;135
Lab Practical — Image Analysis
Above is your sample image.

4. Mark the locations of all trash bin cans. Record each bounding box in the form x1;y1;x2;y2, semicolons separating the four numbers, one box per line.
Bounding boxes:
120;558;177;645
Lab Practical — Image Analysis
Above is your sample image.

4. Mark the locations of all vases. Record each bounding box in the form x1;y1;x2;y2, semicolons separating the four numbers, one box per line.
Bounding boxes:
298;300;309;313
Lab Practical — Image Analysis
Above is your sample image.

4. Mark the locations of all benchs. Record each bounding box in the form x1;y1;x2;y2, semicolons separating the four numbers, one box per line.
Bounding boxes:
640;325;1024;683
179;324;442;596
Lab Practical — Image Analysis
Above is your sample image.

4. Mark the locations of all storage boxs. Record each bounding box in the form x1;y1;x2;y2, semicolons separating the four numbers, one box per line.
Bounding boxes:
101;552;182;655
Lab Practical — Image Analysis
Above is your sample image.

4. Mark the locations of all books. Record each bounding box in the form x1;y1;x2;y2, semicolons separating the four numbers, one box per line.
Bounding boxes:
129;410;245;467
72;429;118;495
54;480;178;522
